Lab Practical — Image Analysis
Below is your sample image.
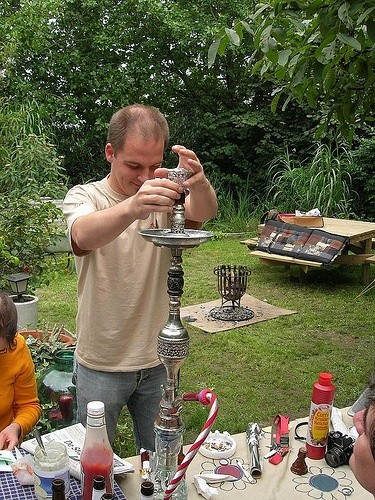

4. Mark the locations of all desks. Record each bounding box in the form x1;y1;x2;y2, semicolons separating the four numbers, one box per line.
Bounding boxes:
258;217;375;285
0;406;375;500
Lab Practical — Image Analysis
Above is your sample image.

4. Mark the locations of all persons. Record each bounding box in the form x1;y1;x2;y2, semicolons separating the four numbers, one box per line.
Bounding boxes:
62;103;217;455
0;290;42;451
348;381;375;496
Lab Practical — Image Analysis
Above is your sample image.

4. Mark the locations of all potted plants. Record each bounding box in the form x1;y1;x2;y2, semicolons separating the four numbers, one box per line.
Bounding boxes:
19;331;72;372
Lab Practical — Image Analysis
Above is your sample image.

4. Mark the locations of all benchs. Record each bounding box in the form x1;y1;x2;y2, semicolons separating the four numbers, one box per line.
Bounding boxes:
240;238;375;273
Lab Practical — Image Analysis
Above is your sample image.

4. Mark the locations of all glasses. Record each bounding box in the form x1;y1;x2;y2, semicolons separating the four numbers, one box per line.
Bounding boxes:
0;338;7;355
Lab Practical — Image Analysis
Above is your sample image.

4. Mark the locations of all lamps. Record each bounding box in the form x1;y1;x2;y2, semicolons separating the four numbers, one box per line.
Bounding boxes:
6;273;32;301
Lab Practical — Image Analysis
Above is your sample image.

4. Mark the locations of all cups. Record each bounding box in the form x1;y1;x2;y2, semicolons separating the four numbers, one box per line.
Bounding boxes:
305;372;335;460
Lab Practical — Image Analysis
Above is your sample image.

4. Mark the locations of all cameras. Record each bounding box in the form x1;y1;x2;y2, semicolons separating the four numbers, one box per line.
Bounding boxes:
325;430;357;468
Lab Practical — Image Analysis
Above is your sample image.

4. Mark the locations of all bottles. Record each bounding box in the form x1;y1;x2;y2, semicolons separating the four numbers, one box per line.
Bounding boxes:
141;482;155;500
51;478;65;500
81;400;115;500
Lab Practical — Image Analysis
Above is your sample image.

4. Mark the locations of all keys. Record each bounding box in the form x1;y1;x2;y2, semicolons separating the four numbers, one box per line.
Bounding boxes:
263;443;284;458
